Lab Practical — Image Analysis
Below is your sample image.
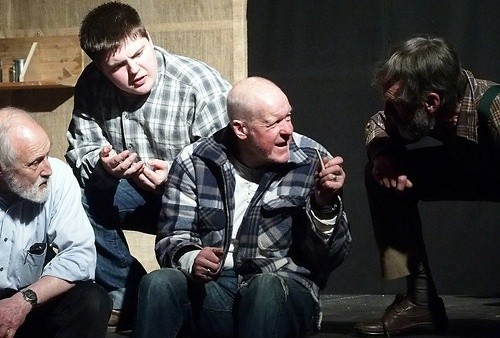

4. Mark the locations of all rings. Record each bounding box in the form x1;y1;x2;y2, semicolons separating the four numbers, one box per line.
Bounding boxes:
331;174;337;182
206;268;211;276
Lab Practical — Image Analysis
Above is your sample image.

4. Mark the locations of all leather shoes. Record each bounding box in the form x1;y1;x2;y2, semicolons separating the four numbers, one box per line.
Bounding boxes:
353;293;449;338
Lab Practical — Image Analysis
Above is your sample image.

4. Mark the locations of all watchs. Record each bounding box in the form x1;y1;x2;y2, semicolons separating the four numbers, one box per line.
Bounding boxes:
20;287;38;311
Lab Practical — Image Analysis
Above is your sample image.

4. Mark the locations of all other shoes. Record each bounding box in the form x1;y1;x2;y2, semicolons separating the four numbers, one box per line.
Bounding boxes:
108;309;136;325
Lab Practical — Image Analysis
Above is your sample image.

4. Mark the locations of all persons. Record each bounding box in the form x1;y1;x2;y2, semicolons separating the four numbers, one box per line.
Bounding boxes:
131;75;353;338
63;1;234;335
0;105;98;338
352;31;500;338
